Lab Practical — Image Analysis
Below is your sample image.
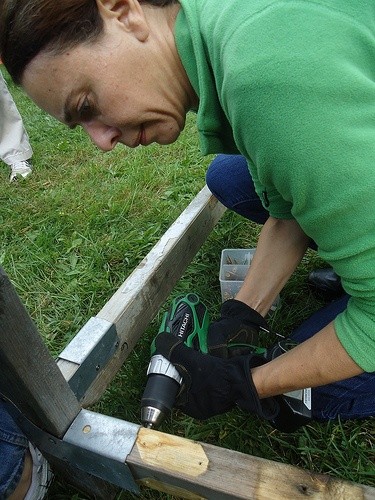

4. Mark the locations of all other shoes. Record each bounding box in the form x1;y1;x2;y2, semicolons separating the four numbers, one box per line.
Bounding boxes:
21;442;55;500
308;265;344;299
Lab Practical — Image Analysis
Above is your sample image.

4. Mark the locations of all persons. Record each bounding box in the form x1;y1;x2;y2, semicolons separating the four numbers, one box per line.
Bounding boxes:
1;0;375;422
0;72;35;182
0;408;55;500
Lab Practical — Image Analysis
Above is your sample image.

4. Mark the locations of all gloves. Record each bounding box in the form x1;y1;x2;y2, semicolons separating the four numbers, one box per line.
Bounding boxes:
191;299;270;358
153;331;282;421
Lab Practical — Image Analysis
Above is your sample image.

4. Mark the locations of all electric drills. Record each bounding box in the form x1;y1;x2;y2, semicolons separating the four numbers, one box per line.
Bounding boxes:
132;289;314;431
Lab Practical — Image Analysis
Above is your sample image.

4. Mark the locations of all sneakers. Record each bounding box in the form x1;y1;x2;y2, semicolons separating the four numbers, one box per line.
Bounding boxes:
9;159;34;183
